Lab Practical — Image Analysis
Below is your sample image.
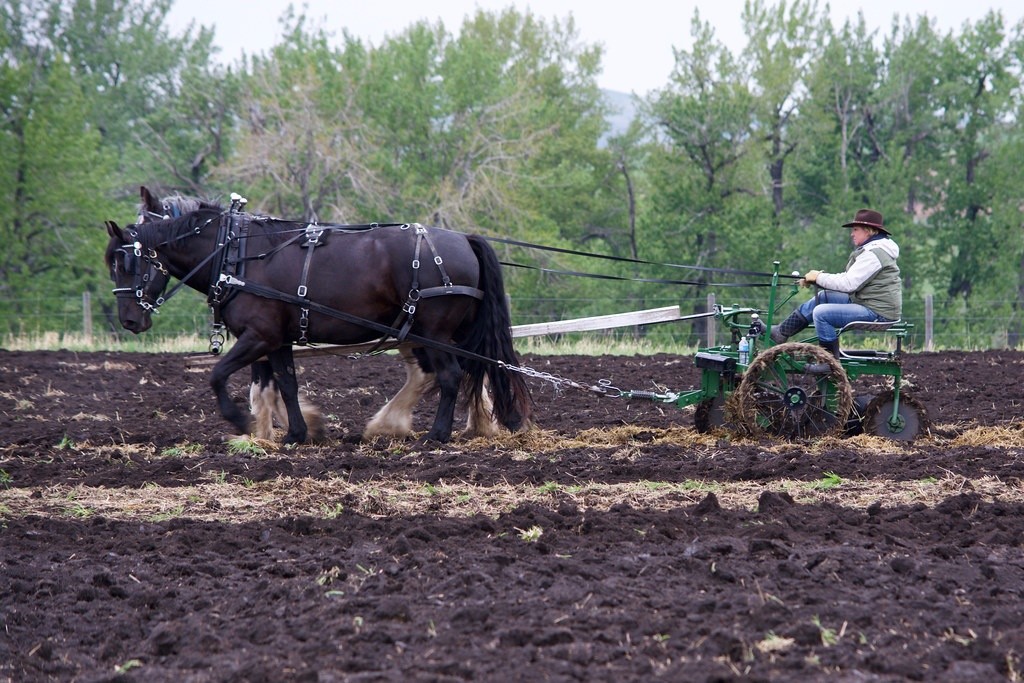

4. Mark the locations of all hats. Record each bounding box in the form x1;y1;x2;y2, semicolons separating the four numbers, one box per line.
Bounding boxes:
842;209;892;236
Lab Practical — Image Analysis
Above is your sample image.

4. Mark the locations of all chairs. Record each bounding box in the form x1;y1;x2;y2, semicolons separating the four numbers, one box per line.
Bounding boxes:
840;316;908;362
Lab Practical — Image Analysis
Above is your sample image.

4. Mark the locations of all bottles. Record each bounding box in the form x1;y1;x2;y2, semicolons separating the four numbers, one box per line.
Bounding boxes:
739;336;748;364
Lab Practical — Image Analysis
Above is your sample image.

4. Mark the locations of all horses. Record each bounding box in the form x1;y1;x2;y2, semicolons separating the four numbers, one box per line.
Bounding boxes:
106;186;539;443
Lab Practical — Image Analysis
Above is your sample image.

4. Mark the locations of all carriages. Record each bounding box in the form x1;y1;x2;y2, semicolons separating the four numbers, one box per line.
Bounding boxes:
105;186;926;449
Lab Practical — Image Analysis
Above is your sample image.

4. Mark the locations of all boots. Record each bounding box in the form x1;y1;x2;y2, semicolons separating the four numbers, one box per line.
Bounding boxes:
804;339;841;374
757;311;810;344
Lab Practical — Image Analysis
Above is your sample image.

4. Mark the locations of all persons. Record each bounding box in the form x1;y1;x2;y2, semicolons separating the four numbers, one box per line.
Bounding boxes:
757;210;902;372
